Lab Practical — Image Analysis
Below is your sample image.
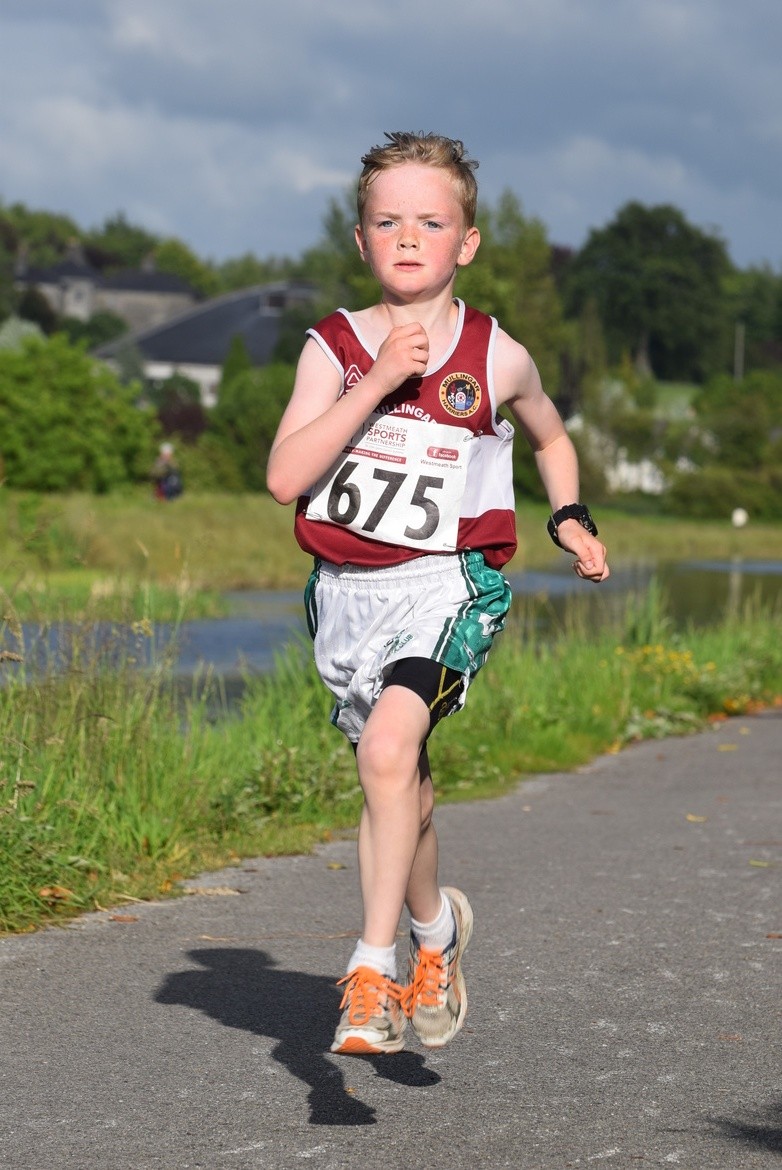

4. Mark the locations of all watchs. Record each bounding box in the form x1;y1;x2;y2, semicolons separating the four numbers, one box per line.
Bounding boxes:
548;503;599;549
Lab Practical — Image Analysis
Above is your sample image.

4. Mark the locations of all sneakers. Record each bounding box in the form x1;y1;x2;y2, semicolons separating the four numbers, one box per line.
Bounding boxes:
330;967;408;1054
409;887;473;1046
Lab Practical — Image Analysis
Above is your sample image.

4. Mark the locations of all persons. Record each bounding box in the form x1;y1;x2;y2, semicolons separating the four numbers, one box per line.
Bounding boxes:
266;131;612;1054
150;444;185;498
